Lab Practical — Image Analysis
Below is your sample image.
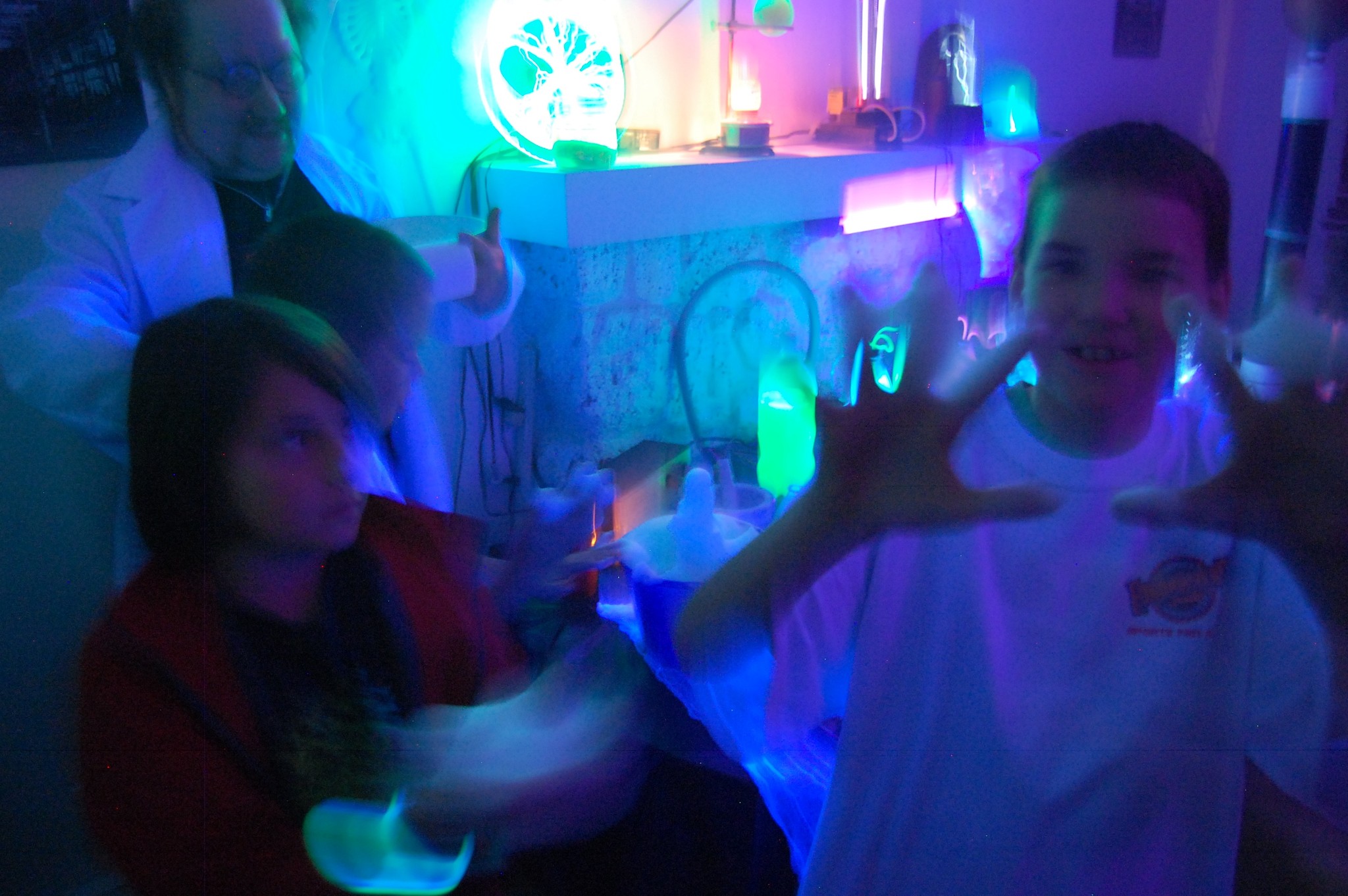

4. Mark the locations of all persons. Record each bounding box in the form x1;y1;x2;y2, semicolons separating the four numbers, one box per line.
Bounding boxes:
240;206;627;626
674;112;1348;896
0;0;529;896
80;296;545;896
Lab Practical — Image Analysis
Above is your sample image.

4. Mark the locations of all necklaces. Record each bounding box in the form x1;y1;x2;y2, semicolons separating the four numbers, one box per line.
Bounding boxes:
210;159;297;224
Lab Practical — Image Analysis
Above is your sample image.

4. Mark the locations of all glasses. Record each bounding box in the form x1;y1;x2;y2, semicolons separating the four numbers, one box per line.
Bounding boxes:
168;50;309;97
